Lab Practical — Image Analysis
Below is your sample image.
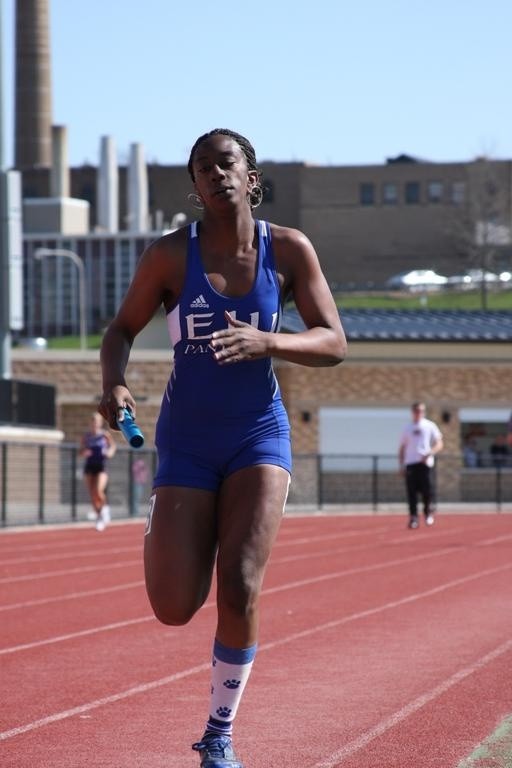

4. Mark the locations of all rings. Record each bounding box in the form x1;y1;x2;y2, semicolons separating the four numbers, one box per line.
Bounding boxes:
115;406;124;413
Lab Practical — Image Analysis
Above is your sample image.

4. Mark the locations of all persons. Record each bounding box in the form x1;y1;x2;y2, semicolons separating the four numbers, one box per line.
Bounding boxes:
398;399;444;532
79;411;118;532
96;127;349;768
459;431;512;470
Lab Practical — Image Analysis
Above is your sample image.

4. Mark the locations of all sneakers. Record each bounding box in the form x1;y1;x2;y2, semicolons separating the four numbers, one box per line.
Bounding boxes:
96;507;109;530
409;513;435;528
192;730;245;768
